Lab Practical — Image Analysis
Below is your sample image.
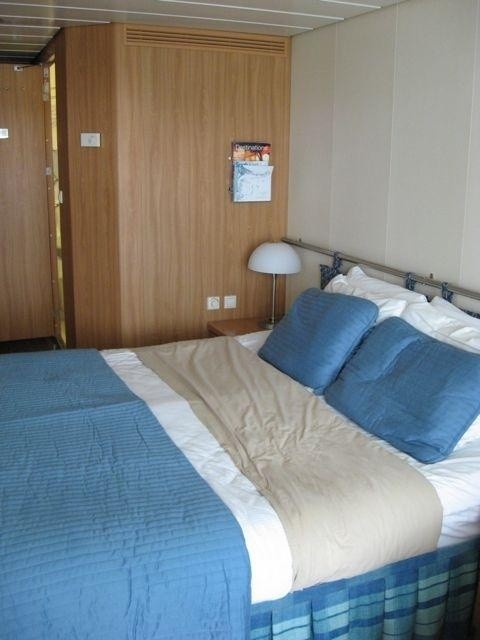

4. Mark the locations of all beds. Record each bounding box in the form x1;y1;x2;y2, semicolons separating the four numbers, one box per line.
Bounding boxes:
1;331;478;638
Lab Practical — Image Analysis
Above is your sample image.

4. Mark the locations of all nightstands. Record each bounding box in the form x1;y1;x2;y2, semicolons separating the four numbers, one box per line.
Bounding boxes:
207;318;266;338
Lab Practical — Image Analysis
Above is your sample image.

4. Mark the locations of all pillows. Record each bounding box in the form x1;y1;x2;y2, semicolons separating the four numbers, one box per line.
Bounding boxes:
330;316;478;464
399;299;479;356
343;266;429;304
324;272;409;323
256;285;380;394
431;294;478;328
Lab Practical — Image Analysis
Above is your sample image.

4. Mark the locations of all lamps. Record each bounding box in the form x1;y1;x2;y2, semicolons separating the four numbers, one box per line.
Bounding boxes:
247;242;301;331
